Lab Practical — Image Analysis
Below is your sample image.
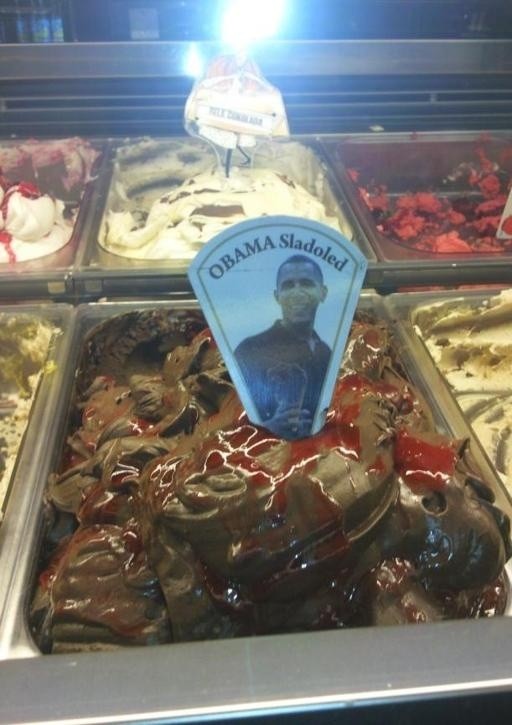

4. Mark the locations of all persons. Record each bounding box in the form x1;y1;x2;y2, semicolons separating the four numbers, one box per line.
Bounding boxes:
234;254;334;443
